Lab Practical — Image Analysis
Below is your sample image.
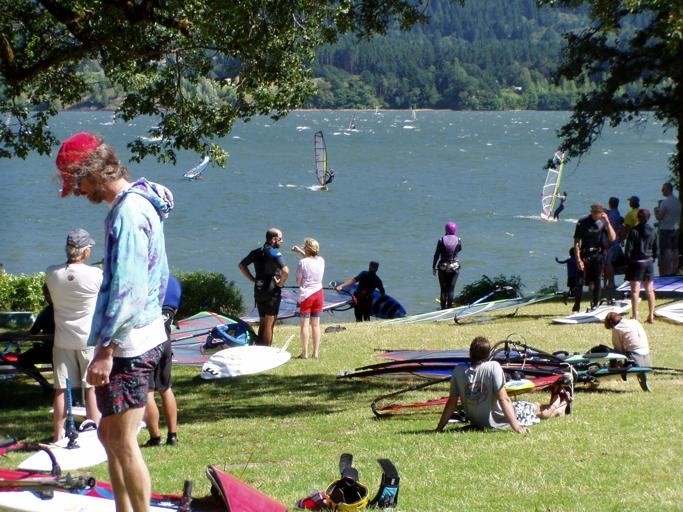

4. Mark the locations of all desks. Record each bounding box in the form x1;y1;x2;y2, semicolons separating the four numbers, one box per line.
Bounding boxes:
1;333;53;341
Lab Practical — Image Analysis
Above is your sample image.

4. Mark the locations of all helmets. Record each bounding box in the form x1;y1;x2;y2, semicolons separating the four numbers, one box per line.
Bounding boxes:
325;476;369;512
447;222;456;234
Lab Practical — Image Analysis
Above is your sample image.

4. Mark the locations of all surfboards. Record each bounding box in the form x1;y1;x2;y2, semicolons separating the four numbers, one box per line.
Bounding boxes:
657;301;683;323
16;430;110;473
200;345;291;381
552;297;642;324
1;491;182;512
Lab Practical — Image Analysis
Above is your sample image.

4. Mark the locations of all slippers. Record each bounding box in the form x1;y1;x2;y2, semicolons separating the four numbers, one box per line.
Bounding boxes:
550;376;572;414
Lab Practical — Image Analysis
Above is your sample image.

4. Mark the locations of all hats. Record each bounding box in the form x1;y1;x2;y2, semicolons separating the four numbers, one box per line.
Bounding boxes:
56;132;101;197
66;228;95;248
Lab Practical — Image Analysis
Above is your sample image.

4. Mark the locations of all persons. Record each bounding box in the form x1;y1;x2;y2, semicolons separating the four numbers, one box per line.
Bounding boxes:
18;281;58;405
145;274;183;449
46;229;104;443
432;221;462;310
56;131;175;512
570;182;683;325
325;165;339;191
292;237;326;359
237;227;289;347
554;192;567;222
602;310;651;379
545;145;565;177
335;258;381;324
435;335;574;435
